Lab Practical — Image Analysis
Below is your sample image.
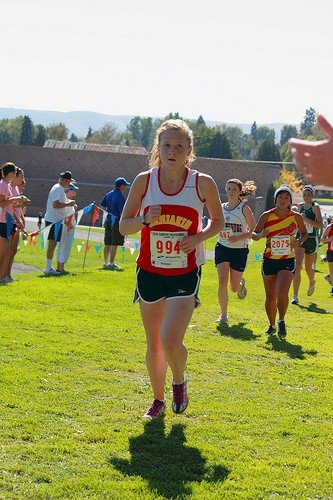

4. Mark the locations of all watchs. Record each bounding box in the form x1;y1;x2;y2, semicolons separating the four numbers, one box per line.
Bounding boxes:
297;239;303;246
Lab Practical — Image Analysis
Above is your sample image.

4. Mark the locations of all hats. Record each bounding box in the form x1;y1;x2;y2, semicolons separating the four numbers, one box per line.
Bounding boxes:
65;184;79;190
114;177;130;186
60;171;76;182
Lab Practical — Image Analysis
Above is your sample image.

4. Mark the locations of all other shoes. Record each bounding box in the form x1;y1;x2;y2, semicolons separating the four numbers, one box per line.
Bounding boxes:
44;267;61;276
264;324;277;336
324;275;331;284
0;275;17;286
277;320;286;338
291;268;296;273
103;263;124;271
291;295;299;304
306;280;316;296
56;269;69;276
215;315;229;323
237;277;247;300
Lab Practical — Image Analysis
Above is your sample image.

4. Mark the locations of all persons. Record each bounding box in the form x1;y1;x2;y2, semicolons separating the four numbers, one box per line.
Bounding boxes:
322;220;333;296
251;184;307;339
44;170;76;275
119;119;225;423
215;179;256;323
99;178;130;272
56;182;77;274
0;162;27;287
291;185;323;303
290;206;333;277
4;166;30;282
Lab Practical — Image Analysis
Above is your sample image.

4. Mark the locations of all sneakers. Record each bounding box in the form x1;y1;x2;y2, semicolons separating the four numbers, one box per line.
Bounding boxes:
170;373;188;414
140;397;167;421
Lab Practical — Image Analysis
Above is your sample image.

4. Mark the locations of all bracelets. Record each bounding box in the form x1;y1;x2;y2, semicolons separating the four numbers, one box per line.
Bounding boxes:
141;213;150;225
